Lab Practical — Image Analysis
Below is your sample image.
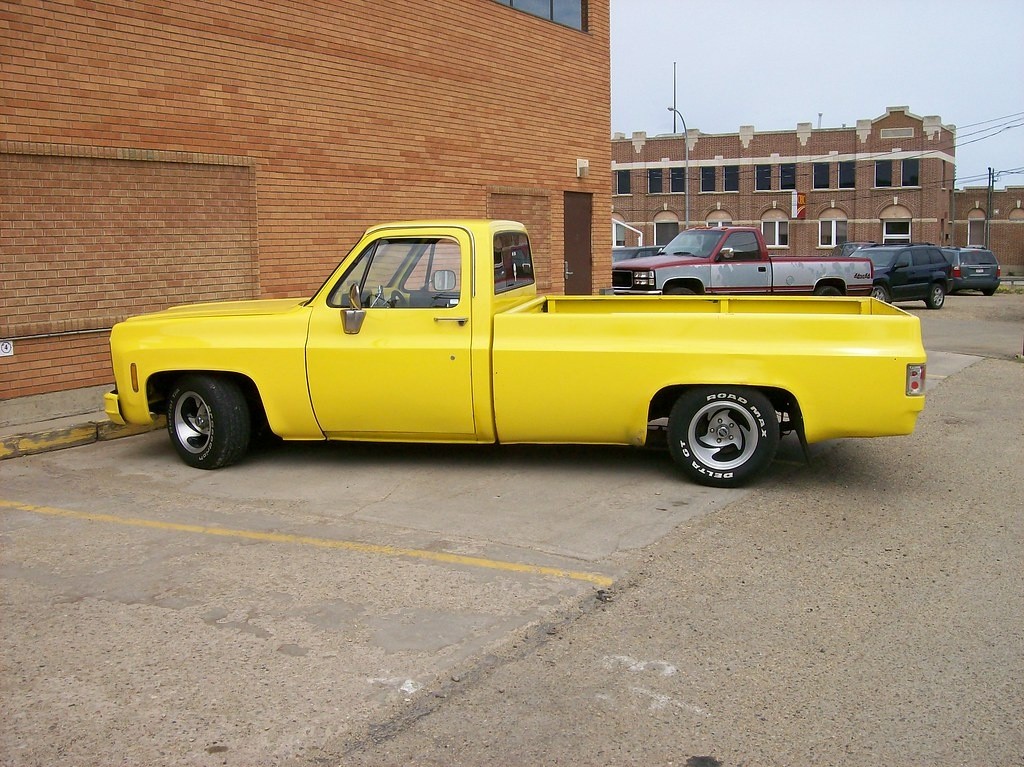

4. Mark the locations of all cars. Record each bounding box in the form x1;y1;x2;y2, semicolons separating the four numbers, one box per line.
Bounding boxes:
611;245;665;262
830;240;877;258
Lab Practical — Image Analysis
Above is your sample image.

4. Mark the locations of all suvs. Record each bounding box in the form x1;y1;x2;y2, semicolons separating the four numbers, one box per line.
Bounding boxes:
847;242;955;310
938;245;1001;296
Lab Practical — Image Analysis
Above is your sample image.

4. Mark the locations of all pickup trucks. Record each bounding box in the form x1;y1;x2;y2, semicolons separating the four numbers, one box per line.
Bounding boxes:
612;226;874;297
493;244;533;293
102;218;928;490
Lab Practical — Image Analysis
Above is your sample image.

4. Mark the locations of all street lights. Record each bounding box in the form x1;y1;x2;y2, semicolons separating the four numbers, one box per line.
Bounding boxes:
667;107;689;229
